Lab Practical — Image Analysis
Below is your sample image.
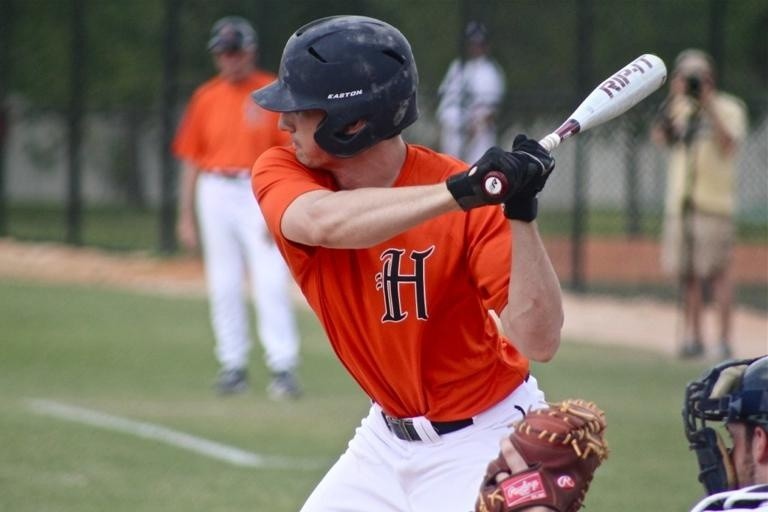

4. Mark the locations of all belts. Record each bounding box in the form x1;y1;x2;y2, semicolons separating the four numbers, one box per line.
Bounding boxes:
381;416;474;441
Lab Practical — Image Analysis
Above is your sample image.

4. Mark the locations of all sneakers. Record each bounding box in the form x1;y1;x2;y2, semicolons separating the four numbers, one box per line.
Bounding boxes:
265;371;300;402
212;363;247;394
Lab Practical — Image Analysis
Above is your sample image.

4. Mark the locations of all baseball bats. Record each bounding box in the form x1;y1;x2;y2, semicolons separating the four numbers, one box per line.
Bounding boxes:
484;54;668;198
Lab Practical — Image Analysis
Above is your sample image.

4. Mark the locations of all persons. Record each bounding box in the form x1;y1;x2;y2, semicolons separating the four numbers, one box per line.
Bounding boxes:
649;49;751;364
244;12;564;512
436;18;504;172
474;348;768;512
174;13;303;401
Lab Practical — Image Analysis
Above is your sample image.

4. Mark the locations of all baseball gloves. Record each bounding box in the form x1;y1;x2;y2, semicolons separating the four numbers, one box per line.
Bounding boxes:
475;398;609;511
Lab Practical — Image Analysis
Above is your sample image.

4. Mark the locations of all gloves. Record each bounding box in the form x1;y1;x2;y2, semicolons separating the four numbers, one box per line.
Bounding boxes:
445;145;537;213
502;133;555;223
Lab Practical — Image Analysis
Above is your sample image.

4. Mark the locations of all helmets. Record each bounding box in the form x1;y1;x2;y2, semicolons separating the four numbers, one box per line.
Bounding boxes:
681;355;768;496
249;14;420;159
206;15;259;56
